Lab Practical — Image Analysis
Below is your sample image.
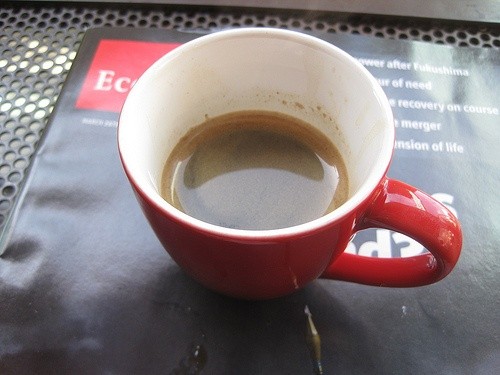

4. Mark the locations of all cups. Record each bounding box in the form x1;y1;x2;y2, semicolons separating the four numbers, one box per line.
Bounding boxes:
120;27;462;299
